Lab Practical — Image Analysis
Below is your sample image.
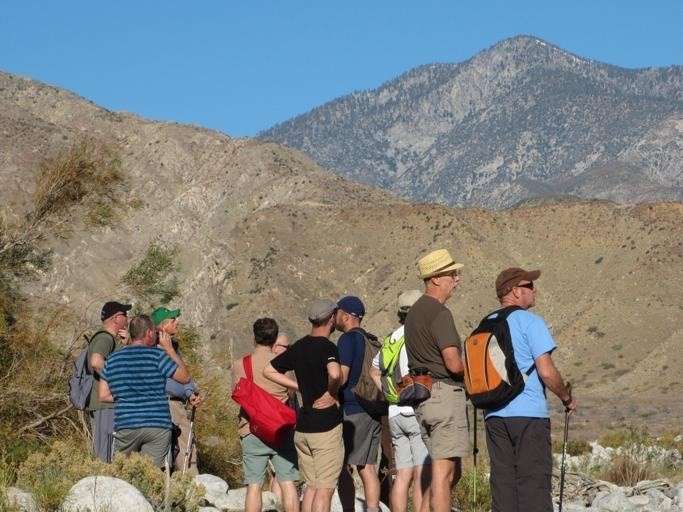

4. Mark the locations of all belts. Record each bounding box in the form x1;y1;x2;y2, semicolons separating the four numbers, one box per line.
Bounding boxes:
167;396;186;404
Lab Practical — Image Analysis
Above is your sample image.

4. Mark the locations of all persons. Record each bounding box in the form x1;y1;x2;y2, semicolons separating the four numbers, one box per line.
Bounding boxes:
481;267;571;512
230;287;428;512
97;314;190;472
149;306;201;475
83;298;132;465
402;247;473;512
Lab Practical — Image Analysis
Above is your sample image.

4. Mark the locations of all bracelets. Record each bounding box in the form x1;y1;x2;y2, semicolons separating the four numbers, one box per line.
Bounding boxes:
561;396;572;406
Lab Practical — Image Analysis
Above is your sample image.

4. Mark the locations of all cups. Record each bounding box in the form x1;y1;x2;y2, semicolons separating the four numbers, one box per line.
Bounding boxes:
156;333;180;351
416;367;429;374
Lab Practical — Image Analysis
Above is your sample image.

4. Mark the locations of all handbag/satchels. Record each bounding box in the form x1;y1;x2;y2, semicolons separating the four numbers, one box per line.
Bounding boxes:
397;368;432;404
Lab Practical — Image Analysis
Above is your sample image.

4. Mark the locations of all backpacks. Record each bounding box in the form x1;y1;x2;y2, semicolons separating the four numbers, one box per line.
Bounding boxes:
67;330;116;410
462;305;526;410
348;330;404;403
231;354;298;449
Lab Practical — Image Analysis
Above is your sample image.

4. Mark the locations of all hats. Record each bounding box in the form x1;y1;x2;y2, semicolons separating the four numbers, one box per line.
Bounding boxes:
336;295;365;320
398;290;424;313
308;295;339;322
101;301;132;321
495;268;541;297
416;248;465;279
151;307;180;326
